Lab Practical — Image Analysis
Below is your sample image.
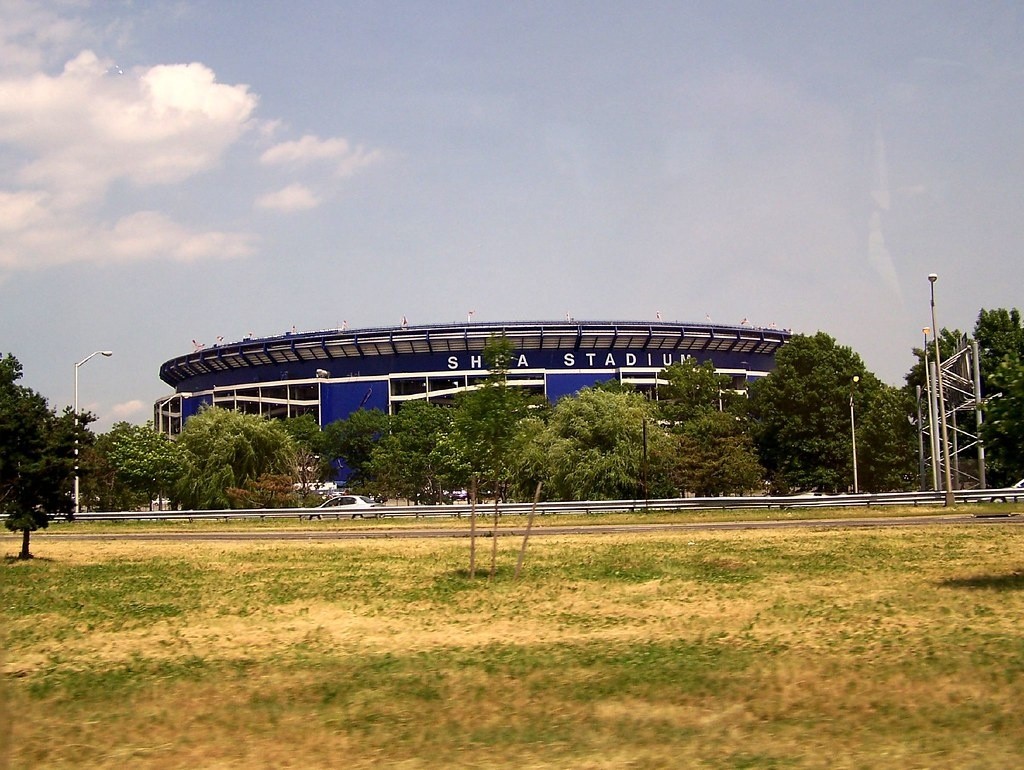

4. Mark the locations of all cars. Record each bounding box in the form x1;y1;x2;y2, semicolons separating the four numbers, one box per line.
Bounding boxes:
977;478;1024;505
302;494;381;521
443;487;474;502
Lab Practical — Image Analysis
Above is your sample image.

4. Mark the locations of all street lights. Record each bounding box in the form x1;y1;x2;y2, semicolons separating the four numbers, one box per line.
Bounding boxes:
926;272;955;510
922;325;938;492
849;375;861;495
74;350;112;518
